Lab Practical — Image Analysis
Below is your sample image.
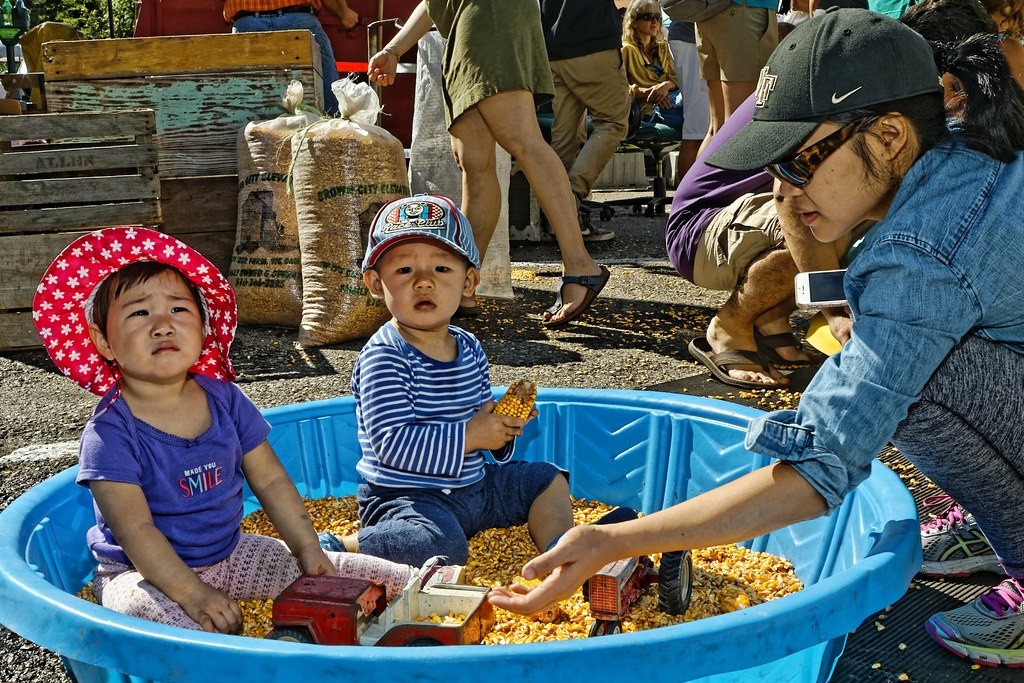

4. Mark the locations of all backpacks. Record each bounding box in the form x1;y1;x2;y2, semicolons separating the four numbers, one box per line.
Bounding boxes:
661;0;732;23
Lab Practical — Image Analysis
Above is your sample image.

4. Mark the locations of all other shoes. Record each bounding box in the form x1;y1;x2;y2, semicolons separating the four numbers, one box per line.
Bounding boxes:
317;531;347;552
545;507;639;574
408;555;466;588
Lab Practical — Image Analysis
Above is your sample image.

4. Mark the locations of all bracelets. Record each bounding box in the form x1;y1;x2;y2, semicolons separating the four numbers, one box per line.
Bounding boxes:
383;48;400;62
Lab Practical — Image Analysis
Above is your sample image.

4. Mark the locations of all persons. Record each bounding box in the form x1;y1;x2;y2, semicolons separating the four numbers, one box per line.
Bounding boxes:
367;0;610;325
490;7;1023;666
223;0;359;119
311;192;639;569
539;0;1024;391
32;227;468;632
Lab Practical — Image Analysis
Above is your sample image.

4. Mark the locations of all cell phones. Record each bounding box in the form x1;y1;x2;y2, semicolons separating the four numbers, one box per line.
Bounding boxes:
793;269;849;310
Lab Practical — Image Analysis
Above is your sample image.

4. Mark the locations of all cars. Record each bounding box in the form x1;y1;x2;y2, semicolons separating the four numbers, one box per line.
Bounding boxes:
0;41;29;102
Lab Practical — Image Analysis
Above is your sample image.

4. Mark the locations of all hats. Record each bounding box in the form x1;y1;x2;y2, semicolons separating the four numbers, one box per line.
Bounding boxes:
702;7;942;172
361;193;481;270
32;228;239;397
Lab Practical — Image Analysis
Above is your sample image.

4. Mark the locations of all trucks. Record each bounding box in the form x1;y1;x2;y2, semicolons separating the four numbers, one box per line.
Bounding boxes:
263;573;497;647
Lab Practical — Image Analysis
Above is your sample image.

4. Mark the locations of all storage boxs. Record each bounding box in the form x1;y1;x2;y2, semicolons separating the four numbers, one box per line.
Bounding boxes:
0;107;163;350
42;27;322;178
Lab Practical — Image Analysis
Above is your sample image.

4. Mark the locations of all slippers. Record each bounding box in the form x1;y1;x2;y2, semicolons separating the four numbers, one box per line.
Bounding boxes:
688;335;790;389
752;324;821;368
542;266;610;328
454;305;482;318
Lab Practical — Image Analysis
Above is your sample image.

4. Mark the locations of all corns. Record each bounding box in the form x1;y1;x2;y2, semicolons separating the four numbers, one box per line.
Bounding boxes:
490;379;537;422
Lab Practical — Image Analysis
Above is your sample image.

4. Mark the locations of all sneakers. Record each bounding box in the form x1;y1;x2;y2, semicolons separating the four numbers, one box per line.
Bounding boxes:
914;494;1006;577
550;211;615;242
925;578;1024;668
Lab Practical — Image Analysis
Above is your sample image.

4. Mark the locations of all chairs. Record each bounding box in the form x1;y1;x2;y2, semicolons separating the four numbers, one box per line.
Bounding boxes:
532;111;615;223
604;100;678;216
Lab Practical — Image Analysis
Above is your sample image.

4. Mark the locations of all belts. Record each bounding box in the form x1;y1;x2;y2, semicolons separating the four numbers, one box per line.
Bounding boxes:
233;5;311;21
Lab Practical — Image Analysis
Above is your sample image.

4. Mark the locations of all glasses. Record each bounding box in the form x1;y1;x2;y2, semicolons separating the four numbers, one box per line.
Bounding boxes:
636;13;661;22
762;114;879;188
